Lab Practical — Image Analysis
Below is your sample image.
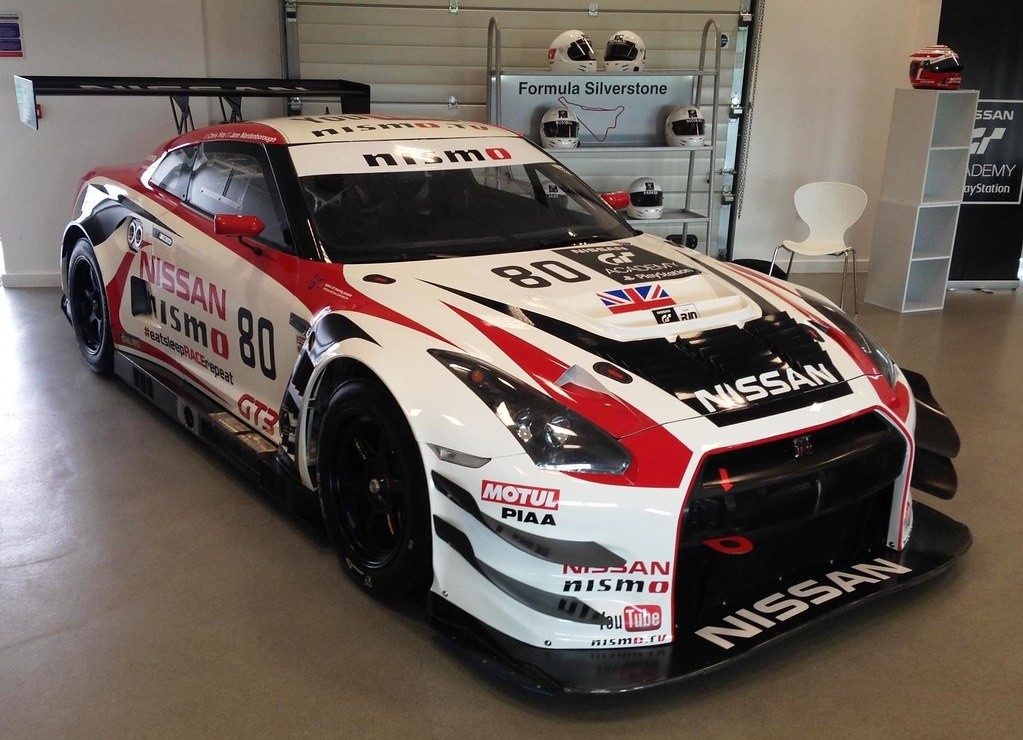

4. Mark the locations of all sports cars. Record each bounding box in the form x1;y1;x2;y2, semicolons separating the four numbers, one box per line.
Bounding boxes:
12;71;976;704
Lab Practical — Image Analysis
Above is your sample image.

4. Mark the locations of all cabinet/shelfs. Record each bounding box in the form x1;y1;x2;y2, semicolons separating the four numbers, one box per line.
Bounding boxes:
864;88;979;313
492;70;709;225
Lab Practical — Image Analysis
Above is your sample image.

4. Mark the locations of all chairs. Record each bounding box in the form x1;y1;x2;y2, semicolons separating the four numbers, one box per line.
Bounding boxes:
768;181;867;314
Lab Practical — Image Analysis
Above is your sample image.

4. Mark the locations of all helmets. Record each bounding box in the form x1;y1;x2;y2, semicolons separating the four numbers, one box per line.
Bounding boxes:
603;31;646;73
547;30;597;72
909;45;962;90
665;106;706;147
627;178;663;219
540;107;579;148
